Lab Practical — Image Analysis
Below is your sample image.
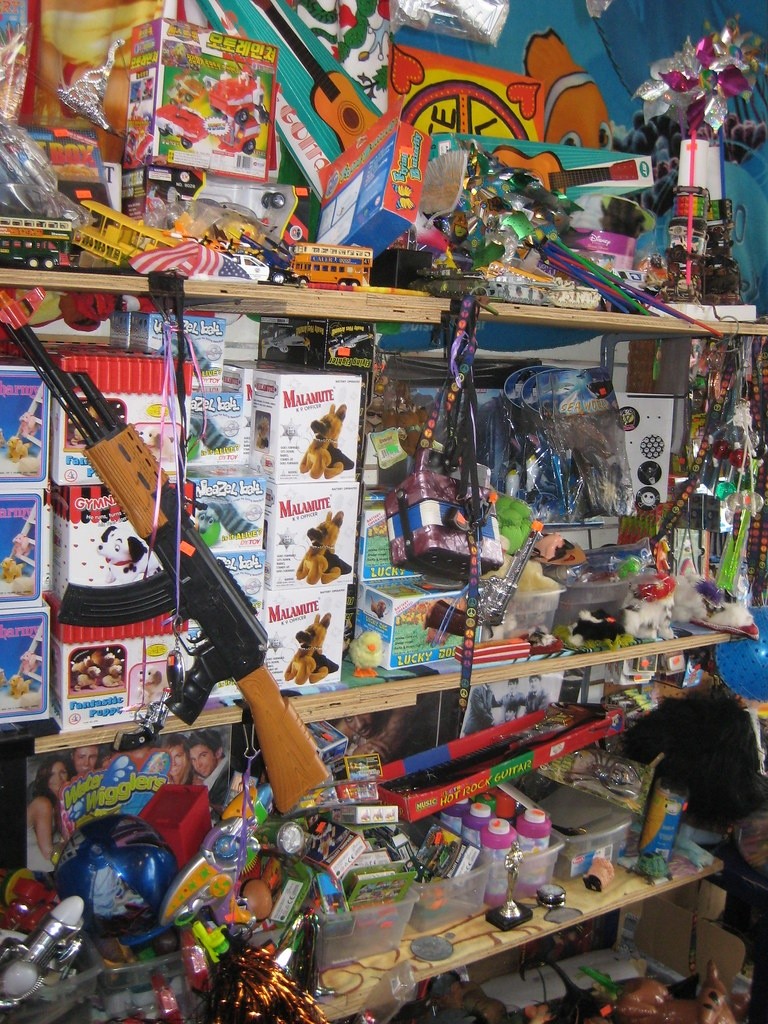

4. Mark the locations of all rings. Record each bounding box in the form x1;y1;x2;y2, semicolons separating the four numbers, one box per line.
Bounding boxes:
369;750;375;754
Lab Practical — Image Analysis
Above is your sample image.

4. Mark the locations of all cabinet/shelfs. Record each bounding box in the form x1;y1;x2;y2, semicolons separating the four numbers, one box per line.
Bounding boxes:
0;269;767;1024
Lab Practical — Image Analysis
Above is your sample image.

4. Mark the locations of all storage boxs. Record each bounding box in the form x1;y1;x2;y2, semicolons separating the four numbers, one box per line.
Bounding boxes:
80;766;633;1019
0;337;688;740
62;15;654;290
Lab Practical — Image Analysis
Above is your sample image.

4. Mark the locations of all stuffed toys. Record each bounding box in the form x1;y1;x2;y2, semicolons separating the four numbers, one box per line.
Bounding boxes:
348;631;383;677
495;496;531;555
568;572;754;653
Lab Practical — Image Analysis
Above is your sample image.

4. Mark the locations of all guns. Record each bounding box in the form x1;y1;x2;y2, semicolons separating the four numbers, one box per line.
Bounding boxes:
1;291;326;814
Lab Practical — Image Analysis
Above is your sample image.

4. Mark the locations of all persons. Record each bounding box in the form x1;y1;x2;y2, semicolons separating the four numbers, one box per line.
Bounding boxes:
334;705;417;765
524;674;552;716
26;745;99;872
165;729;229;805
492;678;525;723
463;681;497;736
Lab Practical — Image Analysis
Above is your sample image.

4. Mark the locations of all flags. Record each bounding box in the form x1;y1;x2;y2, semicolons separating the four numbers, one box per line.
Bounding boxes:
127;240;259;284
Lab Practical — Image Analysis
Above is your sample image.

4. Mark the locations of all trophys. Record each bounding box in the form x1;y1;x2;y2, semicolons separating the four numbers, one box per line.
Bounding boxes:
485;841;532;932
